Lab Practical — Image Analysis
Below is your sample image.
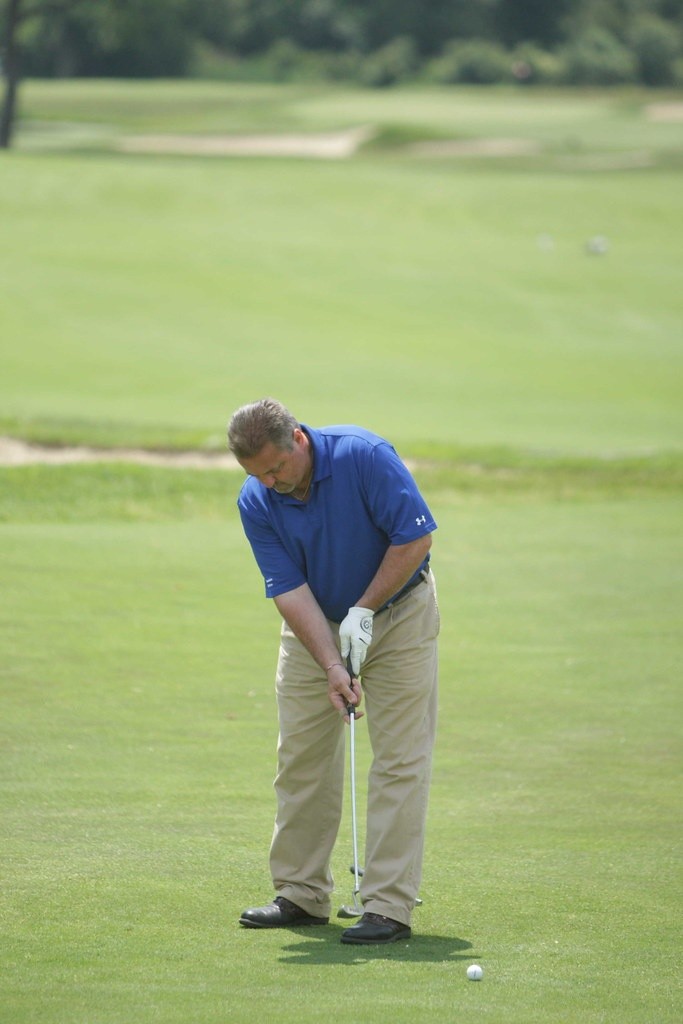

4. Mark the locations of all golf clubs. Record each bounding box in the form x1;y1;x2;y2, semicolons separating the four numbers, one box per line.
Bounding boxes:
335;642;363;920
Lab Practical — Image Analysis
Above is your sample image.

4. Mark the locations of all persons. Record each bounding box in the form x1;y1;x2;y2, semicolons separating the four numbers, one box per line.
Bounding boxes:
226;398;440;946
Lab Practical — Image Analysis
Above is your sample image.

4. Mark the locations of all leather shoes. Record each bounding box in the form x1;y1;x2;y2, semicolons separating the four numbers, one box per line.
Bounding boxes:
239;896;330;928
340;911;412;945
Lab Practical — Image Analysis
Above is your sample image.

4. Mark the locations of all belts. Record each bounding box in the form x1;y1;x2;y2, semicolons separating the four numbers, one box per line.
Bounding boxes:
375;562;430;615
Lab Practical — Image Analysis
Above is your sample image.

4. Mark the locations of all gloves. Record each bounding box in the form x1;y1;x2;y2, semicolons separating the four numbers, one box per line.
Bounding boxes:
338;607;375;676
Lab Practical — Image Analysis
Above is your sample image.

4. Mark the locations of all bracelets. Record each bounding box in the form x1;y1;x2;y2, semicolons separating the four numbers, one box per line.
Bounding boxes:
323;663;343;675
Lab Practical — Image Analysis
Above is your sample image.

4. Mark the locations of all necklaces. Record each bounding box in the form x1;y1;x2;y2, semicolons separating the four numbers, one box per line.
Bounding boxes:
289;468;313;502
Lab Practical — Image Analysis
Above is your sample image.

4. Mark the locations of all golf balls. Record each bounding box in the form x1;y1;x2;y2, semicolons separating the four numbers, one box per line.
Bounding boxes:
467;964;483;980
